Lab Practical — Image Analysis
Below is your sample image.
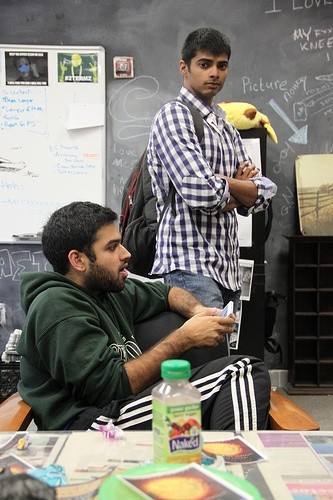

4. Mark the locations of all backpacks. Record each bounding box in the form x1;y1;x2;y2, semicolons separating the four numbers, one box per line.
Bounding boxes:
119;100;204;280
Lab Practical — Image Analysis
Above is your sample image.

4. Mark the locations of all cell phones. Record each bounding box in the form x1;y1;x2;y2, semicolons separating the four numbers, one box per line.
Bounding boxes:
217;301;234;317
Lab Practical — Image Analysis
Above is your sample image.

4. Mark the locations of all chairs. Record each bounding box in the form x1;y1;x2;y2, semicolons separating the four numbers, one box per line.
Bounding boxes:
0;271;320;430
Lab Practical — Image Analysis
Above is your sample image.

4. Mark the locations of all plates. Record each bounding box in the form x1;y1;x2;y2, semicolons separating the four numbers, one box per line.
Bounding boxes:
97;462;262;499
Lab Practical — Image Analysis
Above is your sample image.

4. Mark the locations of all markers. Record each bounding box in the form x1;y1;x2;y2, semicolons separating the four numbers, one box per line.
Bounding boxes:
12;233;40;239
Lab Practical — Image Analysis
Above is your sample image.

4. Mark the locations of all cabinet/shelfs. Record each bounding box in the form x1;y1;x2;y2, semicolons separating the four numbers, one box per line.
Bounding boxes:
285;236;333;397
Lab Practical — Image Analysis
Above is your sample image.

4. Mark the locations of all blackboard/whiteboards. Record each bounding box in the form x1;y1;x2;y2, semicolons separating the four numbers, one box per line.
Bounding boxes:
1;43;107;242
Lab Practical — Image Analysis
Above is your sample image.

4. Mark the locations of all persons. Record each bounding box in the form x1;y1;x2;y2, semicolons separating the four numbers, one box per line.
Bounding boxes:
13;56;45;81
17;202;271;431
146;29;278;309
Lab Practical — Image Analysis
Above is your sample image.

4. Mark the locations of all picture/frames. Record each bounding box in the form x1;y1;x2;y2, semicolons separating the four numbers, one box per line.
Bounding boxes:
3;52;49;87
56;52;99;84
233;129;268;266
112;55;134;81
292;152;333;238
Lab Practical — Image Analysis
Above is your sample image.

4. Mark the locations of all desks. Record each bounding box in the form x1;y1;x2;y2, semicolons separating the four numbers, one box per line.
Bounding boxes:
1;429;333;500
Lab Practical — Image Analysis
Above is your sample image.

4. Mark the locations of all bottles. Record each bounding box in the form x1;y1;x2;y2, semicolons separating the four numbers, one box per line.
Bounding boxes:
151;359;201;464
